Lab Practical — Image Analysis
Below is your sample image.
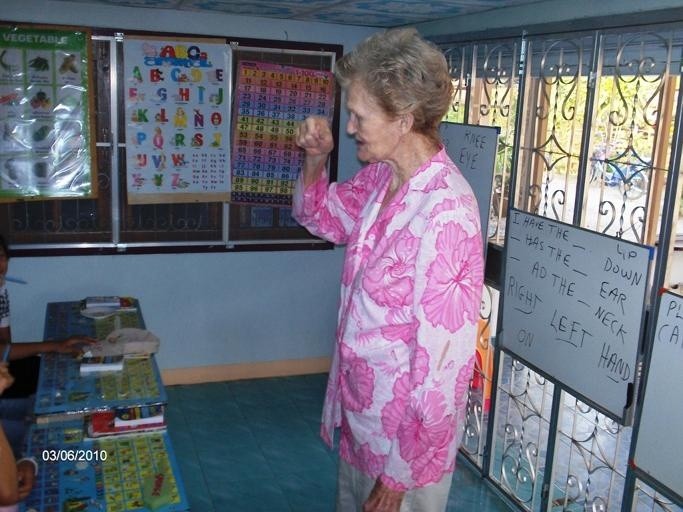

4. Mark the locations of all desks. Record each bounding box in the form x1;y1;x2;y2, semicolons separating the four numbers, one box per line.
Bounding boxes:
19;417;191;512
34;298;170;422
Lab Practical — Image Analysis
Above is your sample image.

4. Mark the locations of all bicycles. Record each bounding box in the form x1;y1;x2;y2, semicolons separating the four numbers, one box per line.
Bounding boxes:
590;142;649;201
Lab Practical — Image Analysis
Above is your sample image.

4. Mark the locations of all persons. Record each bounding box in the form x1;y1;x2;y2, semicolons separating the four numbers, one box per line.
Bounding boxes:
0;238;99;398
292;26;487;512
0;420;37;512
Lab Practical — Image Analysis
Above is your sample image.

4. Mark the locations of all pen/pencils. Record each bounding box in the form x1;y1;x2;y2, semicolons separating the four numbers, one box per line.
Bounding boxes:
2;342;11;361
2;276;27;284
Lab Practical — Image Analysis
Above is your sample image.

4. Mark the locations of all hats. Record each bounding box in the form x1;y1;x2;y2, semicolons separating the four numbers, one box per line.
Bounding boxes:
82;327;159;358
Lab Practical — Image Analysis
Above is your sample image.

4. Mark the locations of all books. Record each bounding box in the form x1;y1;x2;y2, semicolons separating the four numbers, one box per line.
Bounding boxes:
78;294;138;320
79;355;124;372
81;403;167;442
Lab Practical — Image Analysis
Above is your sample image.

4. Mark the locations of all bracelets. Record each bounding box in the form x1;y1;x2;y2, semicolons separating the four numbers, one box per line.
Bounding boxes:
15;455;41;476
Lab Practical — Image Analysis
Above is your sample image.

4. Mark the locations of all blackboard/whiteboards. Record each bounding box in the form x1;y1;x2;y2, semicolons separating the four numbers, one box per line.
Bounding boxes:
436;120;501;265
496;208;652;426
628;287;683;510
